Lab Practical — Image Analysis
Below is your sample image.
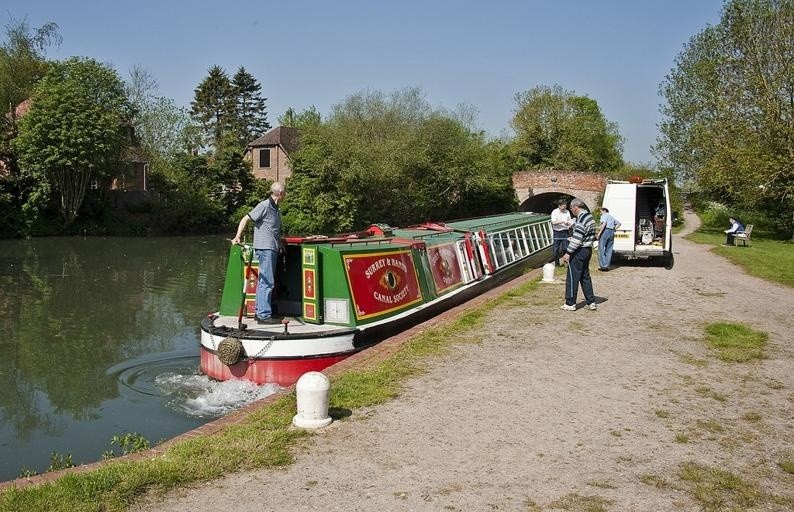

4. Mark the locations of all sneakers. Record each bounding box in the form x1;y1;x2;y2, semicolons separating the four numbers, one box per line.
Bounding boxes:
598;267;608;272
560;304;577;311
584;302;596;310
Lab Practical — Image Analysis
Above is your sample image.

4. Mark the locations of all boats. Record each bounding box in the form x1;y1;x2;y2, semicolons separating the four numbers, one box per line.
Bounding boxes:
201;211;556;389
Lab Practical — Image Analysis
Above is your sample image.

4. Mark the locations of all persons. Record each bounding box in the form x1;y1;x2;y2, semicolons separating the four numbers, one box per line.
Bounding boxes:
551;199;572;266
596;208;621;271
723;218;745;246
231;181;286;324
559;198;597;310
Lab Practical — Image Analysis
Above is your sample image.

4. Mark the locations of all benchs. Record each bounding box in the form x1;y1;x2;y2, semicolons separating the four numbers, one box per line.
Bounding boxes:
731;224;754;247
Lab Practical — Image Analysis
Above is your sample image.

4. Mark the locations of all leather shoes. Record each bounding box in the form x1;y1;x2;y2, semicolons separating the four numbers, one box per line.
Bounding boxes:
254;316;282;324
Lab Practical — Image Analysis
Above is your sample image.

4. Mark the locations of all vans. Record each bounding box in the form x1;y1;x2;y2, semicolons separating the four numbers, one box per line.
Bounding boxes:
600;177;672;260
